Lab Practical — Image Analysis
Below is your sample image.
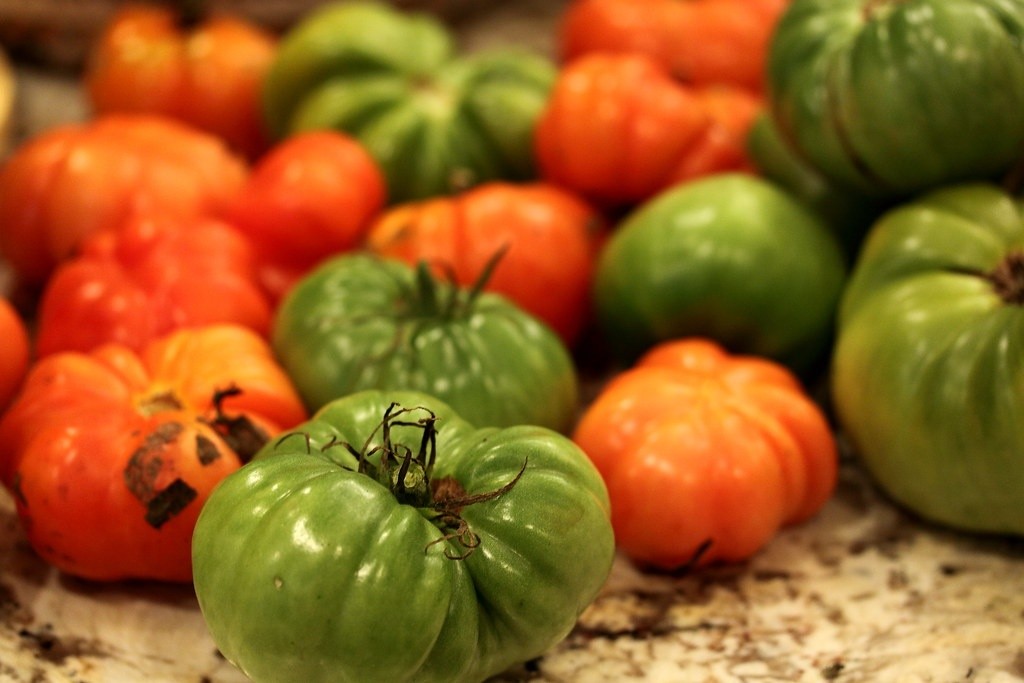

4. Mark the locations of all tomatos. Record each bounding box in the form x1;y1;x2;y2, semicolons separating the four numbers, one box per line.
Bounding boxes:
0;0;1024;683
